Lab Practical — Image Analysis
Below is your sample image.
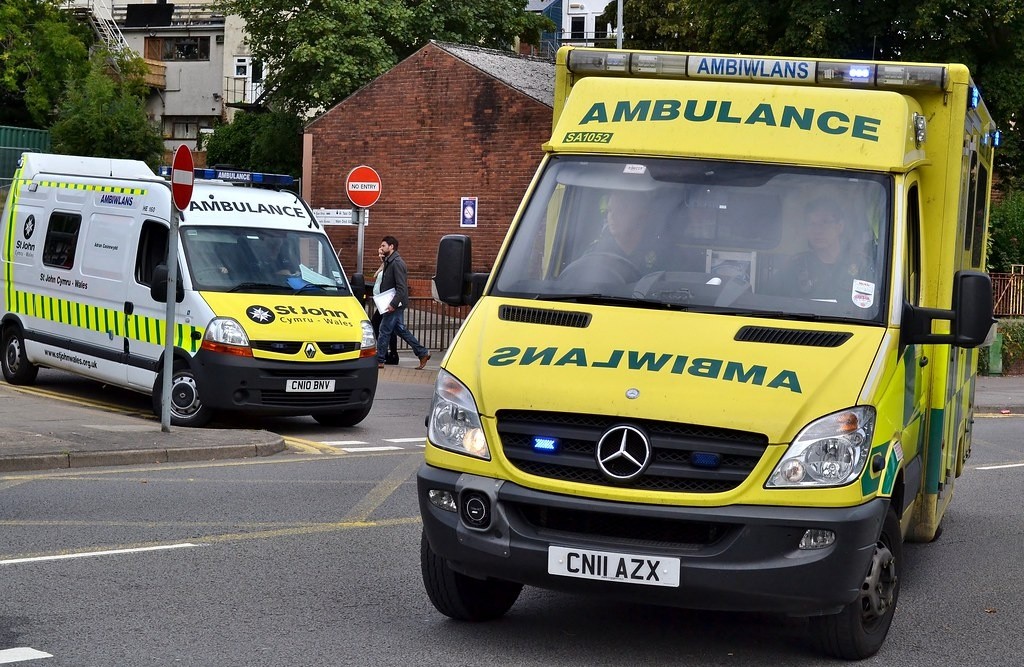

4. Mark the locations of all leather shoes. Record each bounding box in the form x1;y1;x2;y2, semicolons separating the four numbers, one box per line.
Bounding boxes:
415;354;431;370
378;363;384;369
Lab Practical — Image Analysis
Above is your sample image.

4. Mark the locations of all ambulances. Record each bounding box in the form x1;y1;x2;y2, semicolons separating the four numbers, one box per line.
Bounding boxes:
0;151;378;426
417;45;1000;660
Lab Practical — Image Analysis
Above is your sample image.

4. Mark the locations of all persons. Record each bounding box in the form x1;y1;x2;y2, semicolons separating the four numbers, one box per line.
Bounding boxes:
371;248;400;366
771;198;875;303
188;241;229;273
372;233;432;371
585;189;669;284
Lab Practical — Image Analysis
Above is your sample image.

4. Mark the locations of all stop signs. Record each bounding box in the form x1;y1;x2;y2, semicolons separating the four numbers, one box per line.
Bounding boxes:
171;144;195;212
344;166;383;207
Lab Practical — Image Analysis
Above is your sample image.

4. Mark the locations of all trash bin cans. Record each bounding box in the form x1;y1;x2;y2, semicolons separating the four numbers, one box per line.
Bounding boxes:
981;333;1005;375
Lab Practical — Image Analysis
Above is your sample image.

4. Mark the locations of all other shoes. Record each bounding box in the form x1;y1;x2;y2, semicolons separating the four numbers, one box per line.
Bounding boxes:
385;352;399;366
